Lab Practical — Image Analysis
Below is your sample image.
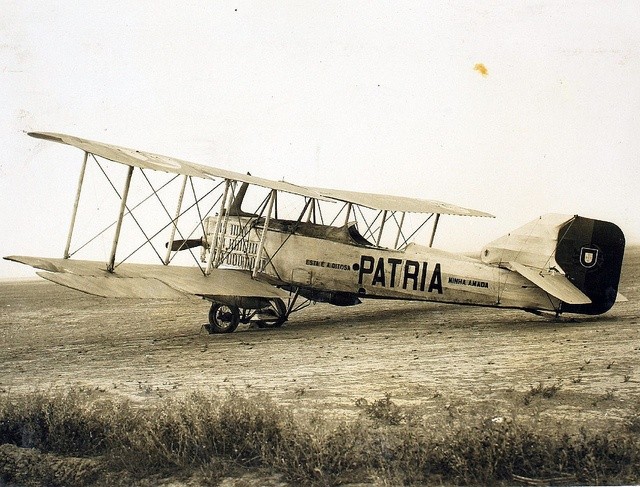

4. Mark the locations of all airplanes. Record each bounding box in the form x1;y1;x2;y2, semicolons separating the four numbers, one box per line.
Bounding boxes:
1;129;629;336
